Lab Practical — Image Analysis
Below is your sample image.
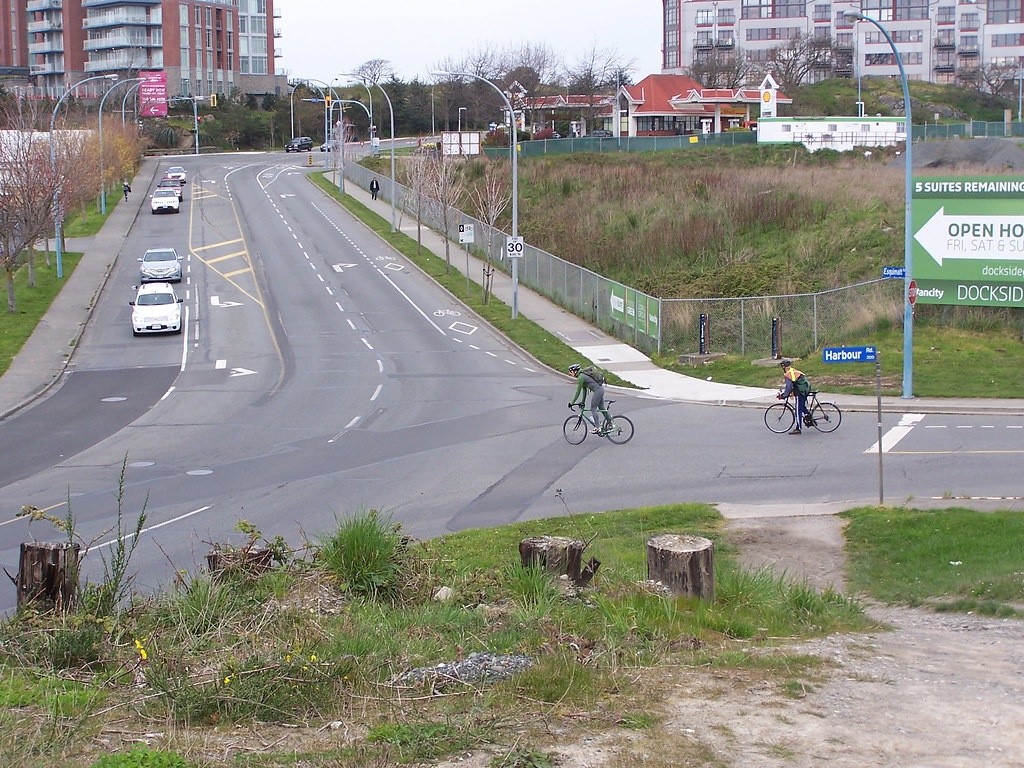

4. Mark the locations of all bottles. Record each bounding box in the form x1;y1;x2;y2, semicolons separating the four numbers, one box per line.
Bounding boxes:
590;416;596;423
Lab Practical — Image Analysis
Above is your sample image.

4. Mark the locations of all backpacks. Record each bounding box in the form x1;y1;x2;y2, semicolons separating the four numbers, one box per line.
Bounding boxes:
578;365;608;387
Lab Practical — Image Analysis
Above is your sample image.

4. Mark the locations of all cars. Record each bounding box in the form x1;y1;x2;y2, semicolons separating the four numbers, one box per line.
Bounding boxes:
129;282;184;337
320;140;337;153
165;166;188;183
149;189;181;214
136;248;184;285
157;178;184;202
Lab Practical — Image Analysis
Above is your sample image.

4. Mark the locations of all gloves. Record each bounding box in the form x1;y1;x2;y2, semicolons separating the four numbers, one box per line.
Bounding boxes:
568;403;572;408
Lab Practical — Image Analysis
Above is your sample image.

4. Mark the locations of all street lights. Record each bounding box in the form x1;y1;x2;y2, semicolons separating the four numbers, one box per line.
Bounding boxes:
329;77;338;151
840;9;913;398
99;77;146;215
122;79;159;126
430;71;519;320
344;78;373;147
339;72;396;234
855;101;864;117
458;107;466;132
50;73;119;279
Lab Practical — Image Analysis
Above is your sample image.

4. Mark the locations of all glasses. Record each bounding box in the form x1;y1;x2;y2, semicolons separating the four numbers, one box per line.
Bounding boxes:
569;371;573;373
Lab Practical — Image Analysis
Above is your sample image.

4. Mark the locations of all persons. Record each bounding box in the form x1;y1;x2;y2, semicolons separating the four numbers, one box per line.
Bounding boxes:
568;364;615;434
370;177;380;200
122;179;129;198
778;360;818;434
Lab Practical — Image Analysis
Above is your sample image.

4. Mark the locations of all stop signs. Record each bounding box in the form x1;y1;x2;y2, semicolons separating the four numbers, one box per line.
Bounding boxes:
907;279;918;306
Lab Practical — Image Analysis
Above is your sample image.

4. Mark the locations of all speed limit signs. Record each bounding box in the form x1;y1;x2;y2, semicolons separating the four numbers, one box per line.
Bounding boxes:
507;236;523;258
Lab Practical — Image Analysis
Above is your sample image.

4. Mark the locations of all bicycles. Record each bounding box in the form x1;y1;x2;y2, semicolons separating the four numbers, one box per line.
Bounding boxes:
764;389;842;434
563;399;634;445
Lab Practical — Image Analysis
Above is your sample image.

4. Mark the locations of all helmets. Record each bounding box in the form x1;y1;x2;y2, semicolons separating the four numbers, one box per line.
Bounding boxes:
780;359;792;367
569;364;581;370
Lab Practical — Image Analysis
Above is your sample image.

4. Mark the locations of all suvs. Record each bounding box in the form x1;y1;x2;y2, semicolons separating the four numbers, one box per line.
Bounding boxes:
582;129;613;138
284;136;314;153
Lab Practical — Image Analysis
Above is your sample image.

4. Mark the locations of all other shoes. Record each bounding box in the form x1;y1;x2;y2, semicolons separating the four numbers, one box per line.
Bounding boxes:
607;424;615;429
788;428;802;435
589;427;602;433
